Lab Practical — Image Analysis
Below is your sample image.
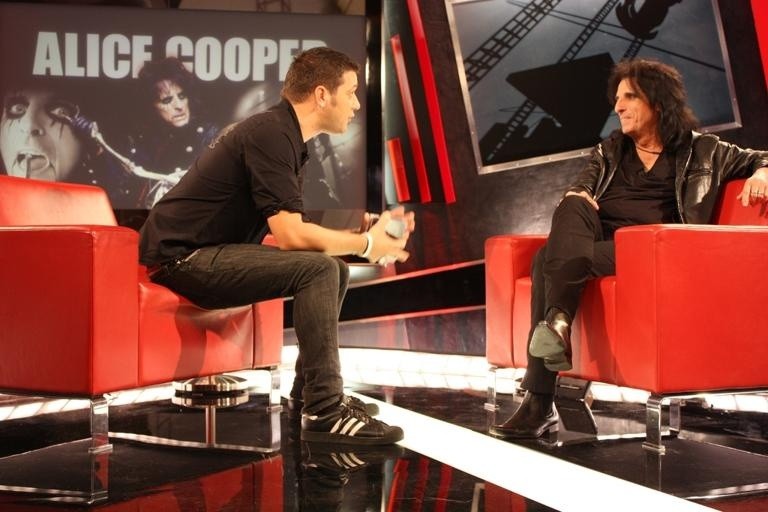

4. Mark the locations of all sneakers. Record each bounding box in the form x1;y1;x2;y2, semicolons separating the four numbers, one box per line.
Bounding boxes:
299;401;404;447
305;443;401;484
286;397;380;426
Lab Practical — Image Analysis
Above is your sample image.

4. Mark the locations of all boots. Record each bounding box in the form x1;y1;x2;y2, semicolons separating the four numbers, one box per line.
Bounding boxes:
528;307;573;373
487;391;560;441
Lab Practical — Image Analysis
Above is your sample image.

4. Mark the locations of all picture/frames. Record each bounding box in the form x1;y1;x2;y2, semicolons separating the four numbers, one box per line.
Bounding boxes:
443;0;743;174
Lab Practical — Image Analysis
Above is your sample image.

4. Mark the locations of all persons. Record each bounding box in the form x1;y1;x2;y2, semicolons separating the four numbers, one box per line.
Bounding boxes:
487;54;767;439
287;446;404;512
139;45;419;445
0;84;98;183
111;54;226;210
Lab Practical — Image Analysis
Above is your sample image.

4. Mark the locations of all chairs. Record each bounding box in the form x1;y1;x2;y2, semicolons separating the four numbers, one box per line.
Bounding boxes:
484;178;765;456
5;454;283;510
0;176;283;455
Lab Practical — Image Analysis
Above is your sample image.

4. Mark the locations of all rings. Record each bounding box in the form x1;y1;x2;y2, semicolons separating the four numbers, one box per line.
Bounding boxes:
751;191;757;195
758;192;764;196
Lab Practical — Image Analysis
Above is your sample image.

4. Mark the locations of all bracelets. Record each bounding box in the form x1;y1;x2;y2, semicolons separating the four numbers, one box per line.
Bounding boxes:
356;231;373;259
